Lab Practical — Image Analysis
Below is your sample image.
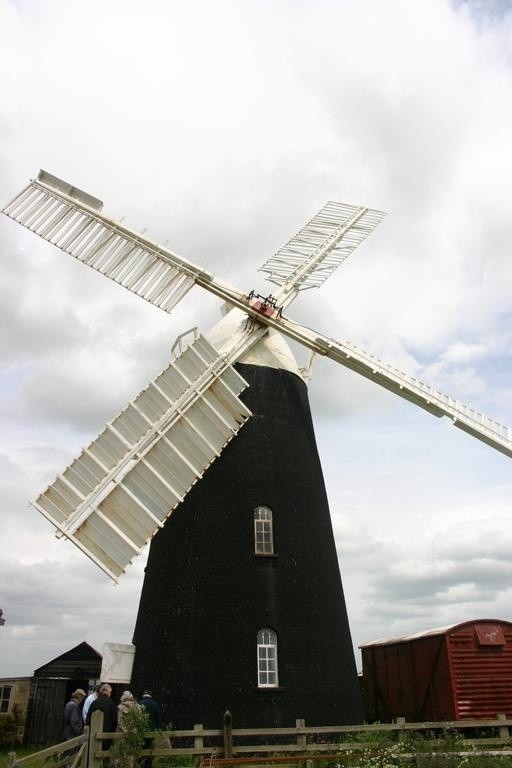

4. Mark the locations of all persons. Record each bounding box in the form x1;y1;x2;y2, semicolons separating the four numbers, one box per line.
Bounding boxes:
60;682;163;768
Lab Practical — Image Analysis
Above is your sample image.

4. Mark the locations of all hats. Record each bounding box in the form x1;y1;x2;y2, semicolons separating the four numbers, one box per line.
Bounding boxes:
142;690;153;697
120;690;133;701
